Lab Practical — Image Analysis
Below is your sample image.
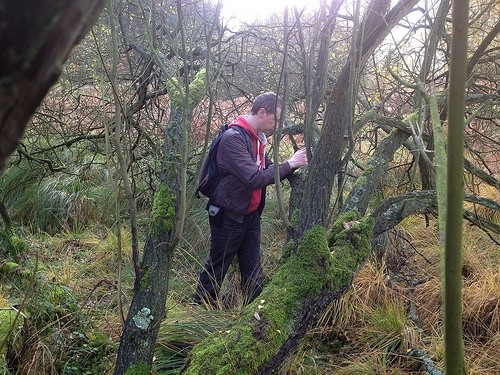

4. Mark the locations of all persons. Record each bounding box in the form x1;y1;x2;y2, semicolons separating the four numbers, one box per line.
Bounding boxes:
192;91;312;305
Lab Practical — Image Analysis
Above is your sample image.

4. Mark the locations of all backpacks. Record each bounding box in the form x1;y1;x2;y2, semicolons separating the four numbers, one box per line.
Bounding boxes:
195;124;247;199
